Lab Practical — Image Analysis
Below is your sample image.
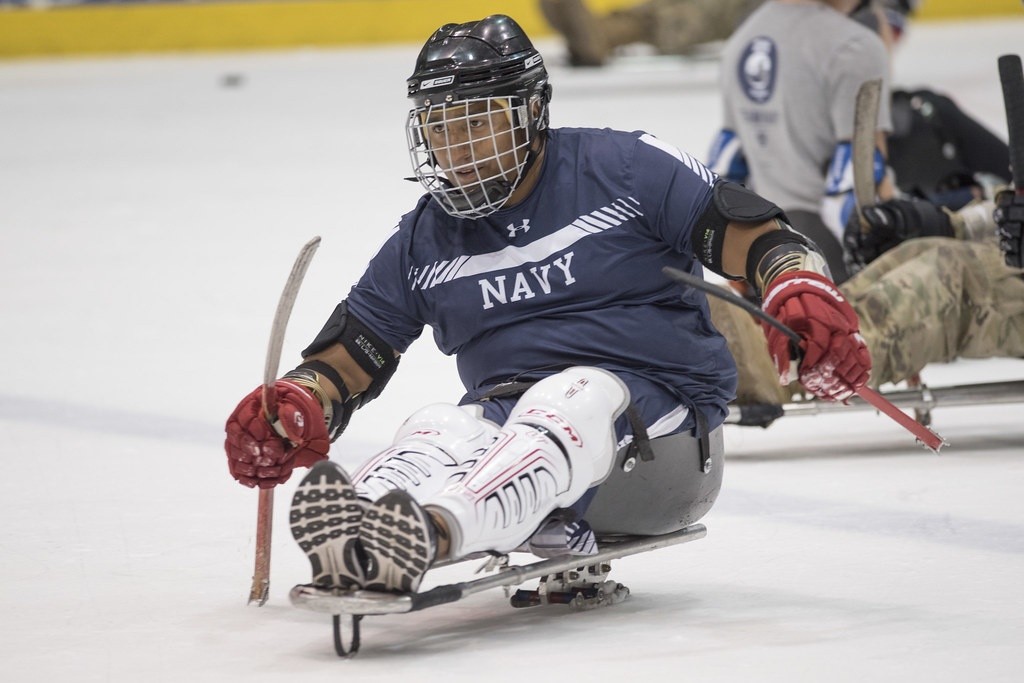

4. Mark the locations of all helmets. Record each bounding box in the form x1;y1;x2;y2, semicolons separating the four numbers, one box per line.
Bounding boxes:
407;14;553;218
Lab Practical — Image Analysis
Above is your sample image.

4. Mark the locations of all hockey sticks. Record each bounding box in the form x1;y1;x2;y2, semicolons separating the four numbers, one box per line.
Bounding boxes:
852;73;886;226
996;57;1024;190
245;238;319;607
655;266;948;454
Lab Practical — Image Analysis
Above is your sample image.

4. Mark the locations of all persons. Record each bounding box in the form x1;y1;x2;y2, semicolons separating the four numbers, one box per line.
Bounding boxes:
225;13;871;595
699;1;1024;405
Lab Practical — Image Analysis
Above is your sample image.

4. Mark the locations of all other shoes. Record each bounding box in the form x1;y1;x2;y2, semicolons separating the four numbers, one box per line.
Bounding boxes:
290;460;366;591
359;489;449;593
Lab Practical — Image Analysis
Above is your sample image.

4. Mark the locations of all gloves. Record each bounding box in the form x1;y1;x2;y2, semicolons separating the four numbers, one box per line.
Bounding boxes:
993;190;1024;268
759;271;872;404
225;379;330;489
844;199;953;269
